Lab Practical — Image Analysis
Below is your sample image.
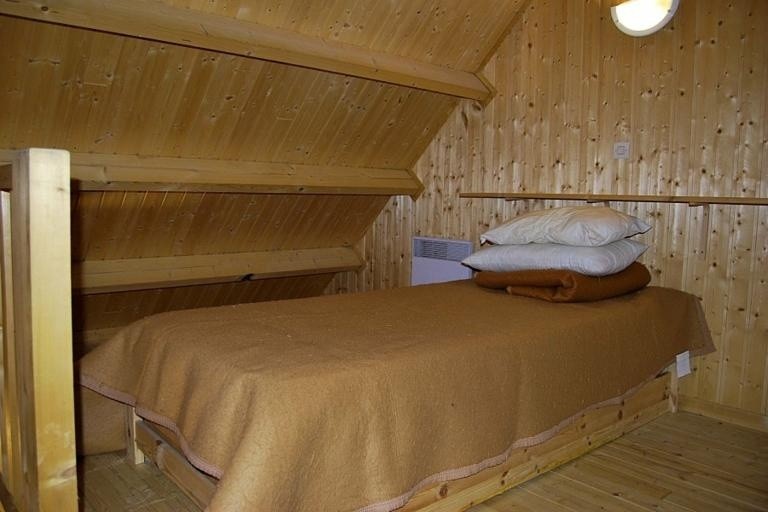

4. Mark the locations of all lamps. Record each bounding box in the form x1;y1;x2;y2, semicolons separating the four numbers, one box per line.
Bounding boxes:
610;0;679;37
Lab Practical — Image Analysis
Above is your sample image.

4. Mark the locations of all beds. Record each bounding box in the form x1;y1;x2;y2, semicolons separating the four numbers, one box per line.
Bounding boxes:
75;280;717;512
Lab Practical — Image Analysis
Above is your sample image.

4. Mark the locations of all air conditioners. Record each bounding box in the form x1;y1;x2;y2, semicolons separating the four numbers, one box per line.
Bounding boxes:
410;235;472;285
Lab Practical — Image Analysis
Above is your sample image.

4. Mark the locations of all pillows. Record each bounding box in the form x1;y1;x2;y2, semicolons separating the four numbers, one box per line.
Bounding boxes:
479;205;651;246
462;237;651;276
